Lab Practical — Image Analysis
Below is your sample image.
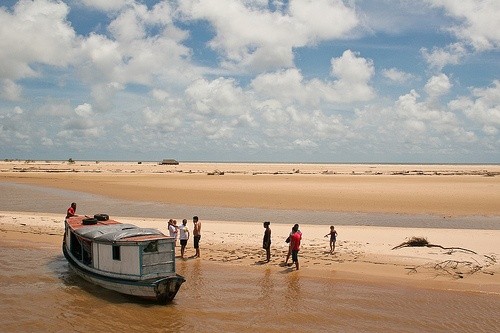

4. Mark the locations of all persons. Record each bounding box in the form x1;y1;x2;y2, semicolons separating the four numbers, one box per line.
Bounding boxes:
173;220;177;231
285;224;302;263
193;216;201;258
324;226;338;253
63;203;78;241
289;227;301;270
263;222;271;263
168;219;178;247
168;219;189;258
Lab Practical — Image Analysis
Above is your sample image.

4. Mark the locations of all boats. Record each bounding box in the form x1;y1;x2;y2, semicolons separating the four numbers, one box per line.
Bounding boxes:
62;213;186;304
